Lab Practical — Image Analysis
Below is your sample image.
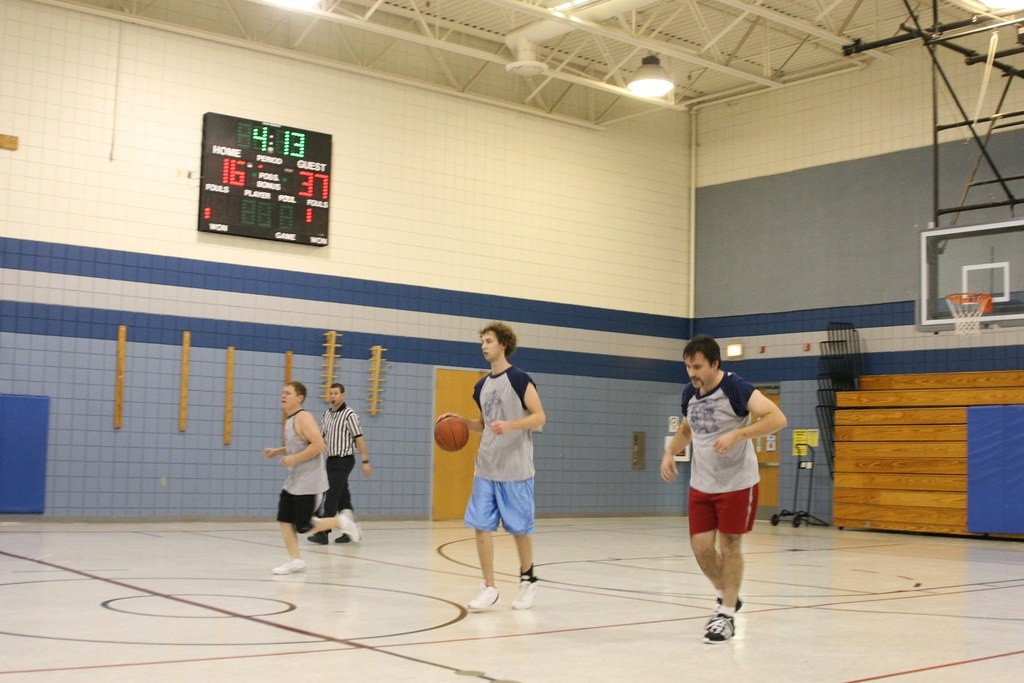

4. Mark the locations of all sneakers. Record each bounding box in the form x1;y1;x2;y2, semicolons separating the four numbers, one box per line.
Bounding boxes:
272;558;305;575
468;579;499;609
513;576;538;610
702;595;743;644
335;533;351;543
336;509;361;542
307;531;329;545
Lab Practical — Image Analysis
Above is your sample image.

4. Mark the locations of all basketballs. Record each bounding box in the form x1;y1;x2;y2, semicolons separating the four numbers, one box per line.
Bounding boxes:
433;417;469;452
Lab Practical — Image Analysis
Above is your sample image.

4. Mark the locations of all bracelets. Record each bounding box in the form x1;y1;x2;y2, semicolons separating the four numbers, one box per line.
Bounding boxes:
362;460;369;464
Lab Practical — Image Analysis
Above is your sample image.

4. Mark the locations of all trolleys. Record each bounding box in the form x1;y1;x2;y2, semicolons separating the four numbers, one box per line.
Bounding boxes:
771;443;829;529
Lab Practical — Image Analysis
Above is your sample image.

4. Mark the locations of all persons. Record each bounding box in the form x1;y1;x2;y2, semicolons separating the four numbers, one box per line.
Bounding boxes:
435;322;546;615
264;381;362;576
660;336;788;645
307;383;372;545
